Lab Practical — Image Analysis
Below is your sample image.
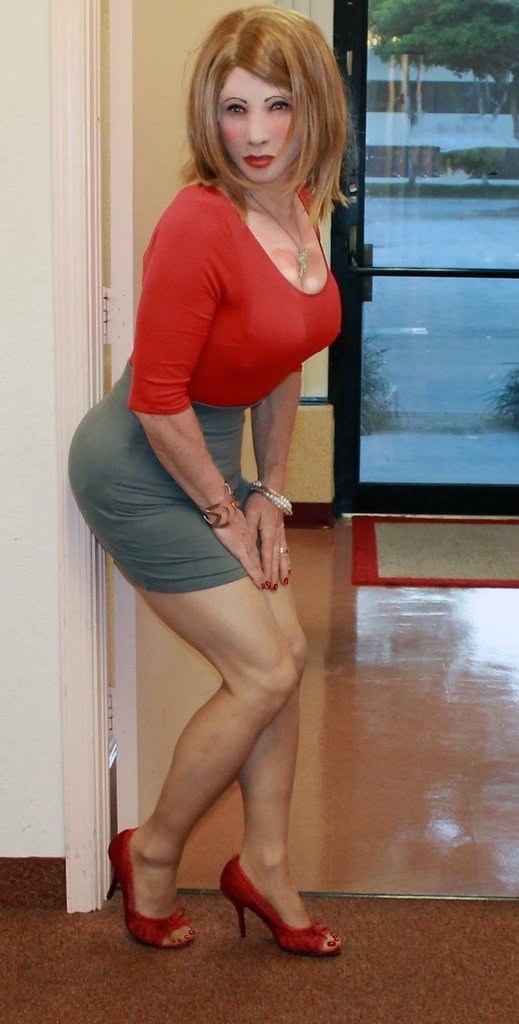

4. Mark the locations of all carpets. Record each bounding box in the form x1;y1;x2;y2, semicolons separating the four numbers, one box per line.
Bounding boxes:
352;515;519;588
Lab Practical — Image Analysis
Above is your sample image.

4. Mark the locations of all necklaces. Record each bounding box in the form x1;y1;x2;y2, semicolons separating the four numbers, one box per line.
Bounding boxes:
241;183;309;278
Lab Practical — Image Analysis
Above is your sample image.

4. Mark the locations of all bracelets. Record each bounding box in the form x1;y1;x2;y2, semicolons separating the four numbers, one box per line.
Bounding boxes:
247;479;293;517
199;480;240;530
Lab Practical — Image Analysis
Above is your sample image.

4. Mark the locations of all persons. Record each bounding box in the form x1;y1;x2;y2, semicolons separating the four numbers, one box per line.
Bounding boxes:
65;4;352;960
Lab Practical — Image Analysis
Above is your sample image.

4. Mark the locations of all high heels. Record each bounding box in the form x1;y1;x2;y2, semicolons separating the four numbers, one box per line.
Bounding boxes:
106;827;193;950
220;854;343;956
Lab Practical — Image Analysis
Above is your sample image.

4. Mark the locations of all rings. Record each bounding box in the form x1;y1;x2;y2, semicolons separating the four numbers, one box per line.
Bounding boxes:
279;547;290;555
